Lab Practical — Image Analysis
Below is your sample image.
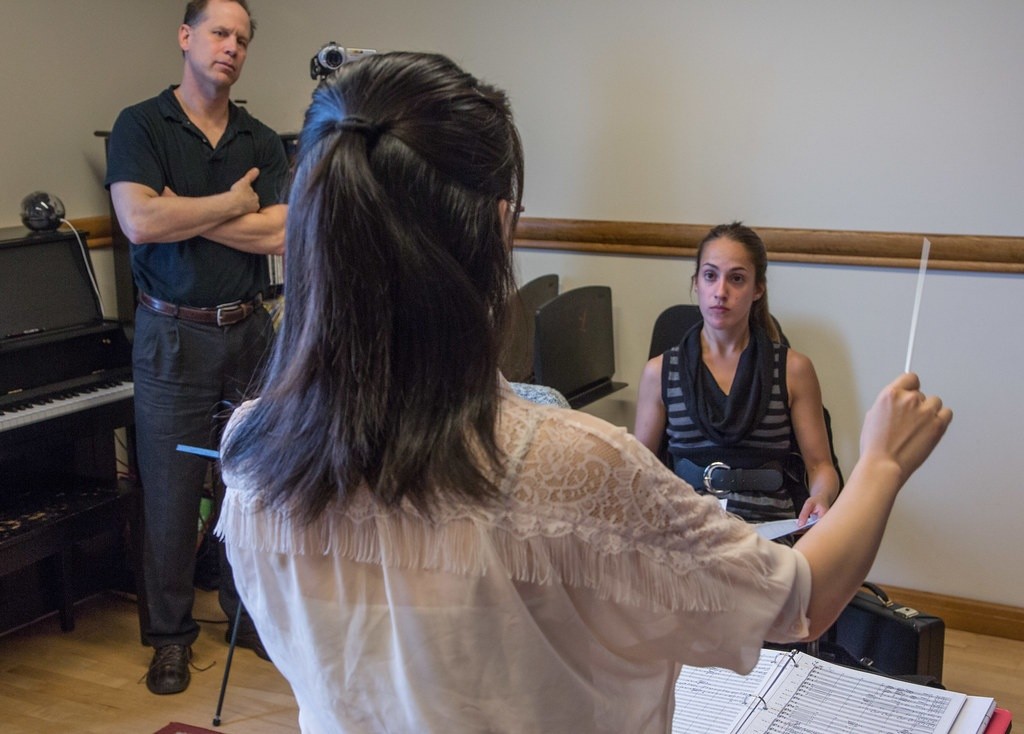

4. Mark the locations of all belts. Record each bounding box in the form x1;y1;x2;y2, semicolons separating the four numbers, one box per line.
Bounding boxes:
669;455;786;495
138;290;262;326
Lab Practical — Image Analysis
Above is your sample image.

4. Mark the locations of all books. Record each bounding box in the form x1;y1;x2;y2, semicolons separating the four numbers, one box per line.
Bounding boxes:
670;648;1012;734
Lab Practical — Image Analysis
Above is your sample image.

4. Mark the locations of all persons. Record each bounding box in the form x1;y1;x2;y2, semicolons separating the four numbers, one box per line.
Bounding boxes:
632;222;841;547
218;49;955;734
104;0;292;695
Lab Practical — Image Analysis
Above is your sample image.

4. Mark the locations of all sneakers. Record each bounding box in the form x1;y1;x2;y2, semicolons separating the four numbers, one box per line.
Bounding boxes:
226;627;271;662
137;644;217;696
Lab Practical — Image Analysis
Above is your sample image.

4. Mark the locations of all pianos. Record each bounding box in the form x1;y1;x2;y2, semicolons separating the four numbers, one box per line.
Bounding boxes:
0;227;146;649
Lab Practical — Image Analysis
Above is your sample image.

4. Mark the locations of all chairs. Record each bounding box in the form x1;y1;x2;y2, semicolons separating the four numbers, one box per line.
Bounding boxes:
492;273;845;655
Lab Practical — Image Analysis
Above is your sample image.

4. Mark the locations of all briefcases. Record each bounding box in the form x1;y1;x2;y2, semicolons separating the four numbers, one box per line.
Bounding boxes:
788;581;946;687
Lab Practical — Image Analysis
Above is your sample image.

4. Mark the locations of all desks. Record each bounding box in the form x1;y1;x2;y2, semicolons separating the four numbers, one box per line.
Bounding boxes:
176;422;243;724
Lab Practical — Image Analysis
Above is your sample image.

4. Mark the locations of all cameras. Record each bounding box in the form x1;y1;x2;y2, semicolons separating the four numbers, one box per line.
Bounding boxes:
310;44;377;83
